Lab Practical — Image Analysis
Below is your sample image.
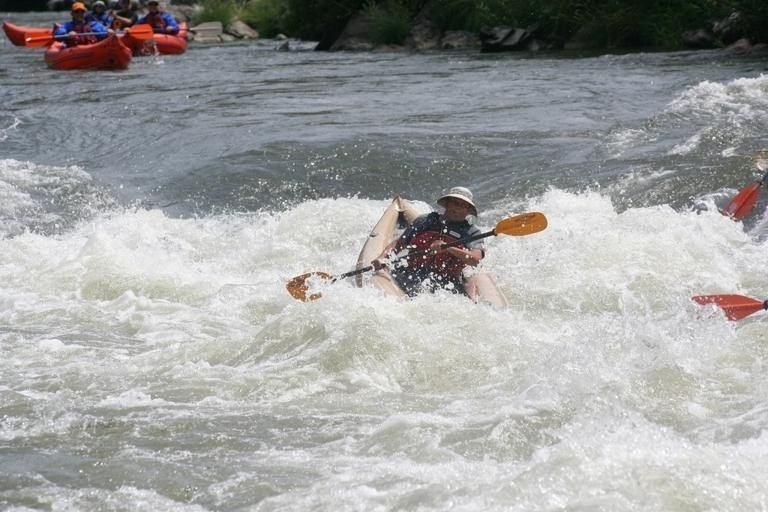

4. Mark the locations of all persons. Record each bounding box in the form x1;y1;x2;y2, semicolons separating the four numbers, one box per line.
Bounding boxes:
108;0;138;32
135;0;179;35
85;0;112;27
371;186;485;302
53;2;113;50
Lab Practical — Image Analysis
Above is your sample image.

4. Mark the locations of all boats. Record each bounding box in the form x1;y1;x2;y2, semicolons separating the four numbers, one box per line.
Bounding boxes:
2;21;56;48
353;194;506;315
44;27;132;72
129;22;189;55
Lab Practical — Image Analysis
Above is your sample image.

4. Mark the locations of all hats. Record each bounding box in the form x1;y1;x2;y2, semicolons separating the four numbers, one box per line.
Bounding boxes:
91;1;105;11
70;2;87;13
436;185;479;219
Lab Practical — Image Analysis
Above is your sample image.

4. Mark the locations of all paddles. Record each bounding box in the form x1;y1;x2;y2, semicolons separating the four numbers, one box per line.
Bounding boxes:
285;212;547;303
25;24;153;48
723;168;768;219
691;295;768;320
